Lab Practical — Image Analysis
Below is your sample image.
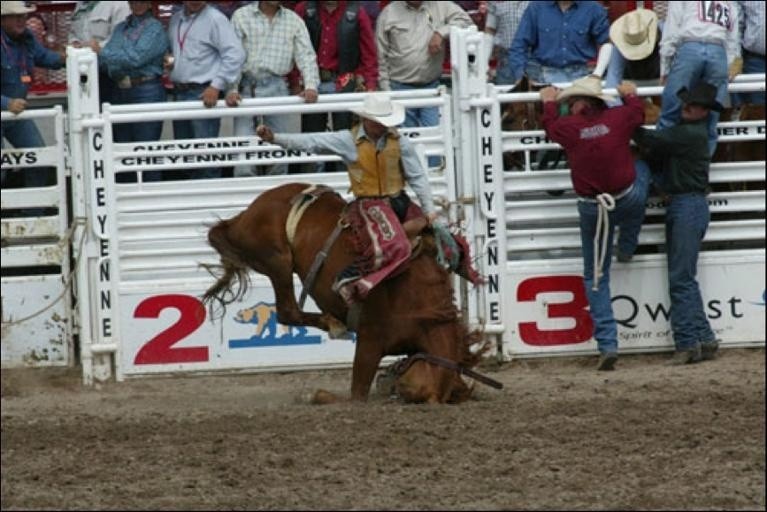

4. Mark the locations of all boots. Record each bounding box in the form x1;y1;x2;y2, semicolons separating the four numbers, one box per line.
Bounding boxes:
330;255;371;303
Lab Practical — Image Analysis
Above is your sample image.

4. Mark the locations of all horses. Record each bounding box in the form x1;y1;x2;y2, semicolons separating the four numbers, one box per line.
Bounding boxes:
195;182;510;405
500;74;766;255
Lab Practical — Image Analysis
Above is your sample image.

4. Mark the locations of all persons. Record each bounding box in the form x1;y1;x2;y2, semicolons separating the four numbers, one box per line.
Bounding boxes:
617;79;725;371
538;73;661;372
1;1;766;218
255;92;447;309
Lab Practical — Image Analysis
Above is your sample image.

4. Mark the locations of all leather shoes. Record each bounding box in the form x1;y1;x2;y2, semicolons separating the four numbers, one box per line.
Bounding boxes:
595;352;616;371
663;340;703;365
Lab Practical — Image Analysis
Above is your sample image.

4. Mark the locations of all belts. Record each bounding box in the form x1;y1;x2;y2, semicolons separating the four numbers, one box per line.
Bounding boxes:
114;75;158;88
167;83;209;93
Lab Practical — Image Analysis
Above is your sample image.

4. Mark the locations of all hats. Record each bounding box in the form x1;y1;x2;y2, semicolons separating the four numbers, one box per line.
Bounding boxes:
347;94;406;127
609;8;658;60
1;0;37;15
555;76;616;103
676;82;726;113
